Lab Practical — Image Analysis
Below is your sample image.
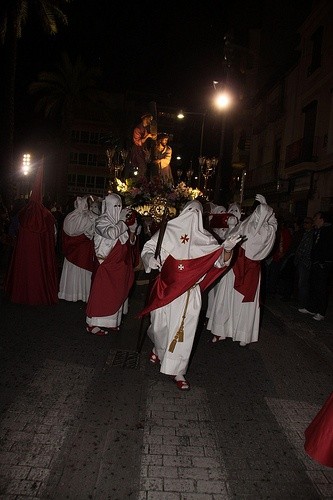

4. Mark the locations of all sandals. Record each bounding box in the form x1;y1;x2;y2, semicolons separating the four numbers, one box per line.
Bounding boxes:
86;324;108;336
104;326;120;331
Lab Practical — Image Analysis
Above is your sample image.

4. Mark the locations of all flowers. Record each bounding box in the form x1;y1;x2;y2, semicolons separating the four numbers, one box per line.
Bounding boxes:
109;177;203;207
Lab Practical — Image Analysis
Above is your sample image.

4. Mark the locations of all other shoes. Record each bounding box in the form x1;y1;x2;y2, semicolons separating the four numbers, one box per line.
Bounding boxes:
211;334;220;343
313;313;325;321
298;307;315;315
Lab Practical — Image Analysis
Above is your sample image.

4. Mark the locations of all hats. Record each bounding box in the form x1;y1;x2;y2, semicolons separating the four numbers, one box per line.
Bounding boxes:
137;111;152;119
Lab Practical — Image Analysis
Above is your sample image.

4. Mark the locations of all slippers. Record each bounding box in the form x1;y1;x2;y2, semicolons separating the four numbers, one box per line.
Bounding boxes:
149;349;160;364
174;376;190;391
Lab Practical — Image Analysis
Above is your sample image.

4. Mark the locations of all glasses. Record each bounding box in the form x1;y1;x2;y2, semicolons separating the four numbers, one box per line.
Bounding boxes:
303;222;311;224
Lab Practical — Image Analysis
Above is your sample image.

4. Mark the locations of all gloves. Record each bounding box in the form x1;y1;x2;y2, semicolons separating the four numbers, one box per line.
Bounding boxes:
120;208;131;221
256;194;267;205
221;233;242;251
149;255;161;270
128;218;137;233
228;217;237;229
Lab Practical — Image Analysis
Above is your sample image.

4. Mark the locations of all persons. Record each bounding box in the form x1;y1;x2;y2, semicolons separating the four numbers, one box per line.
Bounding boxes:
127;112;155;188
260;212;303;326
150;131;175;194
298;210;332;320
294;215;316;311
3;188;62;307
0;192;103;252
57;193;99;305
138;199;243;391
226;201;246;234
84;192;136;336
206;204;227;241
204;193;278;348
